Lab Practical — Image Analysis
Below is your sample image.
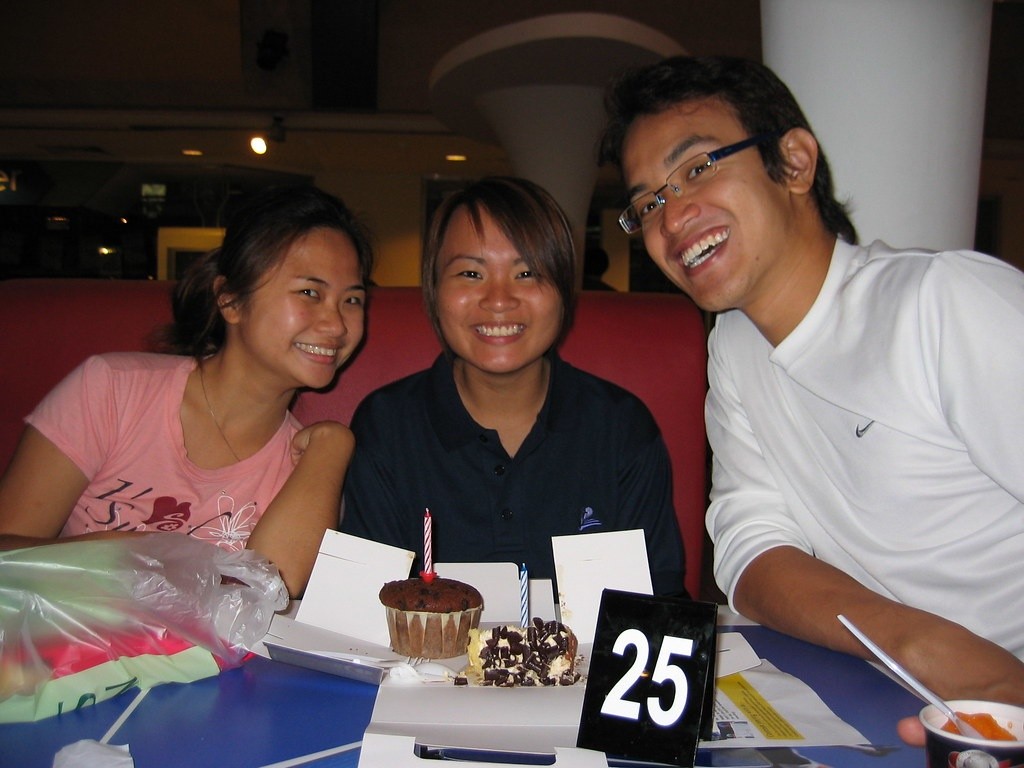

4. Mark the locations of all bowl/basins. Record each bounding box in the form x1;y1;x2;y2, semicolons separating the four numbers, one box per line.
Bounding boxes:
919;697;1024;768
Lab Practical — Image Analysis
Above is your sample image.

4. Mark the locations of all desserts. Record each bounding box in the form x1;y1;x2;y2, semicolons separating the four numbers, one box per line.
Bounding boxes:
381;575;580;687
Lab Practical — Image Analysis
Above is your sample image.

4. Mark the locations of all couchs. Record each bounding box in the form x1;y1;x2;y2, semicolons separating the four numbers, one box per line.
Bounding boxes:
0;276;706;612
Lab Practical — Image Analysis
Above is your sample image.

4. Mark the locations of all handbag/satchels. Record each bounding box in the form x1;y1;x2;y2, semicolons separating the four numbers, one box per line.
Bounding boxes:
0;532;291;722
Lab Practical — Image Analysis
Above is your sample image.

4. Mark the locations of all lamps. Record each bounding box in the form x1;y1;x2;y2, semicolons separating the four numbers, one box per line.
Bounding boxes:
249;116;287;155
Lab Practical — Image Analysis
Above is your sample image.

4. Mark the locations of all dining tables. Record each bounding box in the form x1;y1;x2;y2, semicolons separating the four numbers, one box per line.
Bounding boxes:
0;622;929;768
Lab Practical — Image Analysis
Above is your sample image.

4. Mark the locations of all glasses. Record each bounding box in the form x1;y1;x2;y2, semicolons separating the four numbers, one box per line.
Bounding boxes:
618;137;763;236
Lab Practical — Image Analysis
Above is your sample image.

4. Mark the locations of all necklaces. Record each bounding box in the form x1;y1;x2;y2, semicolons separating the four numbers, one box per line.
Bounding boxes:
199;360;244;463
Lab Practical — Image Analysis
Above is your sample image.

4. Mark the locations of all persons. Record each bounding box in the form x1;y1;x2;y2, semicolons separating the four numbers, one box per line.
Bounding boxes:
0;184;369;598
597;52;1024;709
338;171;694;598
